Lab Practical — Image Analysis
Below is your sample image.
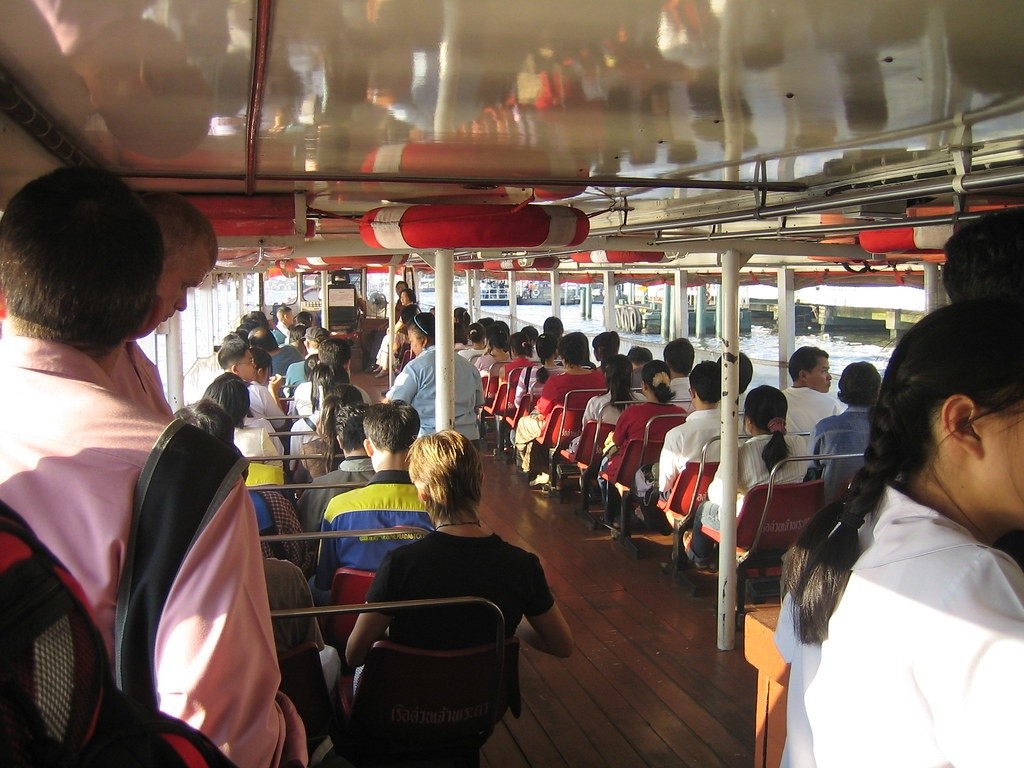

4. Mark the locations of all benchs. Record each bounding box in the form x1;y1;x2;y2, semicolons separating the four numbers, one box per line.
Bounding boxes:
573;397;693;527
535;387;644;502
478;360;564;449
394;347;415;377
686;452;865;631
646;431;812;578
586;410;746;534
506;380;545;466
258;528;431;667
271;595;523;768
483;365;592;462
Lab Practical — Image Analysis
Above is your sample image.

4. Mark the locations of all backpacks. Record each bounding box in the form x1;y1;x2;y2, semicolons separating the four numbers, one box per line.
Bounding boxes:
0;417;249;768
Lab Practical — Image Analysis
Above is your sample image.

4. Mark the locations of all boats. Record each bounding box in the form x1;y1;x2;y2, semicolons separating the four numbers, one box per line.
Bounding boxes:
350;269;752;336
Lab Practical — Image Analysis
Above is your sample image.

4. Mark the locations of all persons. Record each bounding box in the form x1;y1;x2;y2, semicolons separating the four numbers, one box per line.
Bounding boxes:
310;398;436;604
110;192;218;419
289;364;349;470
263;557;342;702
297;402;374;532
344;429;572;736
454;308;880;576
174;399;304;567
296;385;364;495
0;164;310;767
382;313;485;450
777;294;1023;768
371;281;421;379
218;276;372;432
941;206;1024;303
203;373;278;458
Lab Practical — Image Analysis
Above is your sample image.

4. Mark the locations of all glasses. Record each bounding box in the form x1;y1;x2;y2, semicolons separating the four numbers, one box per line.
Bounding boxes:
236;358;254;366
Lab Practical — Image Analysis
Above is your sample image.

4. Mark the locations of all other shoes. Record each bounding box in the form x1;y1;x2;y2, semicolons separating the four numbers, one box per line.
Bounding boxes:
373;367;381;373
684;530;710;569
375;370;388;378
529;472;550;486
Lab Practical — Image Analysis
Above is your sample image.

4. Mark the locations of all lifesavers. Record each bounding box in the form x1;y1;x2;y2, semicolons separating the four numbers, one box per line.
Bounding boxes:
859;223;967;254
360;143;588;180
413;250;665;273
359;204;590;251
275;254;409;273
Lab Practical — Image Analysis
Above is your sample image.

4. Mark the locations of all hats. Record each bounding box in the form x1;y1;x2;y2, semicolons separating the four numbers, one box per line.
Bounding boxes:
302;326;330;343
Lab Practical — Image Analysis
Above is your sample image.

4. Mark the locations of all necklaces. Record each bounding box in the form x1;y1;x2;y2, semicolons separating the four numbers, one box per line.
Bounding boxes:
437;521;481;529
